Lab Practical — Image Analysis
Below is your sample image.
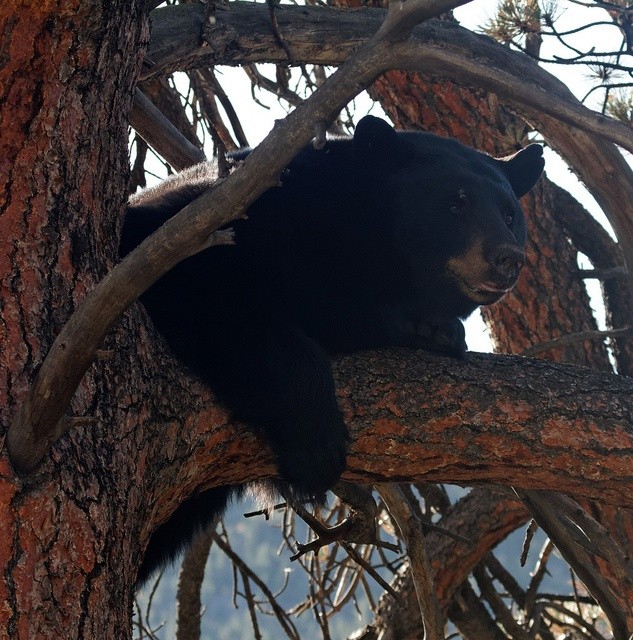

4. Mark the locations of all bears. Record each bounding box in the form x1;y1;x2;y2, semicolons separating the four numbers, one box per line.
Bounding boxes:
115;114;545;593
